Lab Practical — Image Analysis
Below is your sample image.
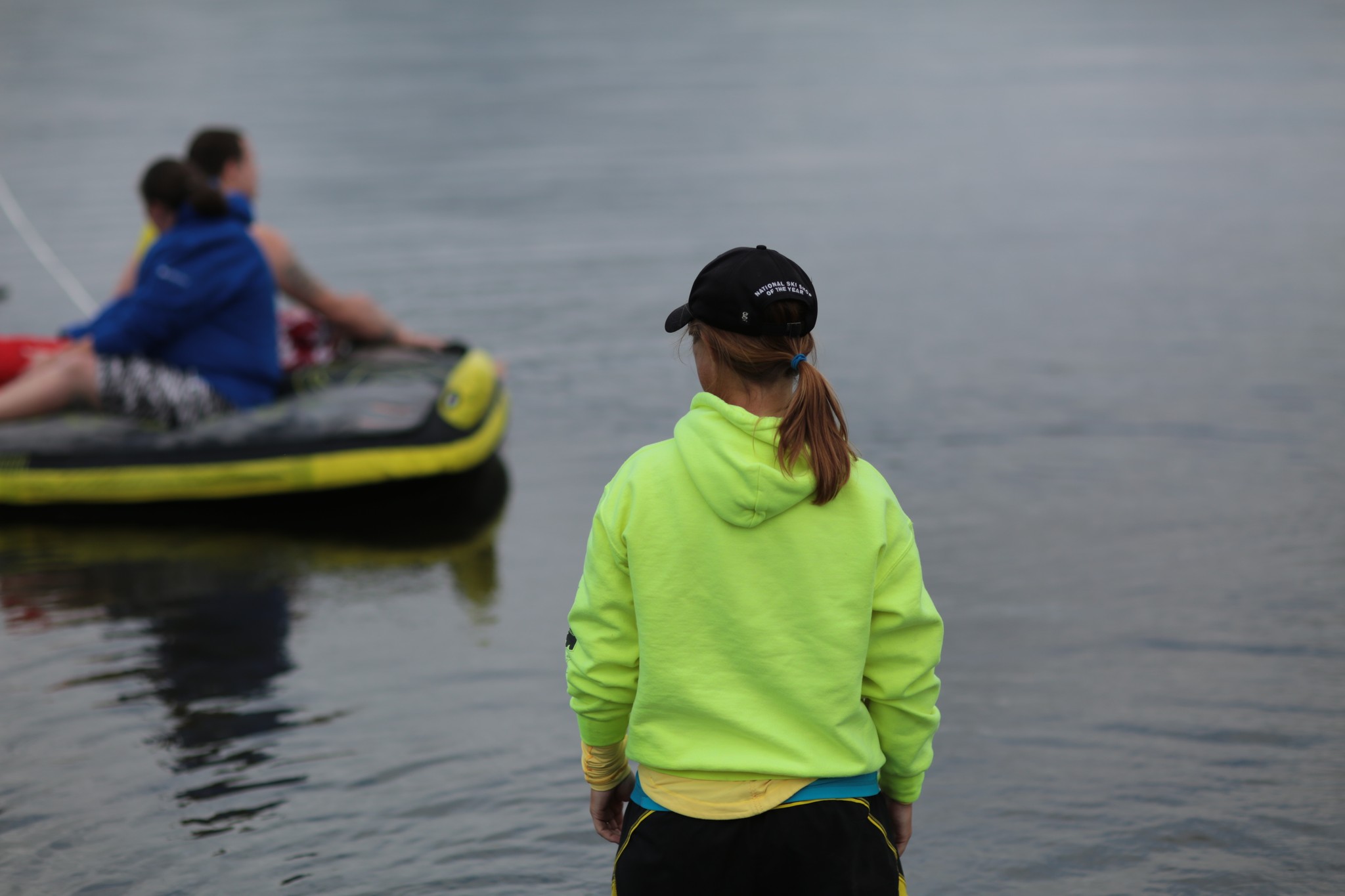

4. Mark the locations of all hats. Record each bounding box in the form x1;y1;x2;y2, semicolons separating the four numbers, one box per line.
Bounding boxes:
663;247;822;340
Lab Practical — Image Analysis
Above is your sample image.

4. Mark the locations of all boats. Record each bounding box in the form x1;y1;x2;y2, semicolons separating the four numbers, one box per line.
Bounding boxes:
0;332;516;513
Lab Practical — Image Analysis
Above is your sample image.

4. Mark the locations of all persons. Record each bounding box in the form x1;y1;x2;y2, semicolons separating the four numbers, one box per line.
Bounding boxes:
564;244;944;896
0;159;286;418
109;129;510;369
